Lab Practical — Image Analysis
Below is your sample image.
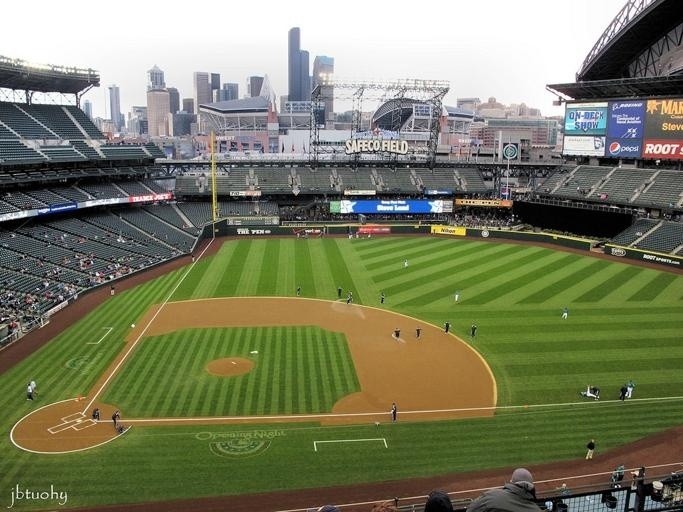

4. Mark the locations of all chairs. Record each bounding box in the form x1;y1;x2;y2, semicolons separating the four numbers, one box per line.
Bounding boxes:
174;163;488;236
1;104;198;351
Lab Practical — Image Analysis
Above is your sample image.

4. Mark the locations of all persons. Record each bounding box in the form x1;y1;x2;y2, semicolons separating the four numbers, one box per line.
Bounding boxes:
0;162;683;511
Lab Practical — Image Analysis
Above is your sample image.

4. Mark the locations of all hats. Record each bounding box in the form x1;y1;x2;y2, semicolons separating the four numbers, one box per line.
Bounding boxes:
511;468;533;484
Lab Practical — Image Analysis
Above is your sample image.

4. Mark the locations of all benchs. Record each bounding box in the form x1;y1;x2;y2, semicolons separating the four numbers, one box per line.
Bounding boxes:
529;161;682;260
